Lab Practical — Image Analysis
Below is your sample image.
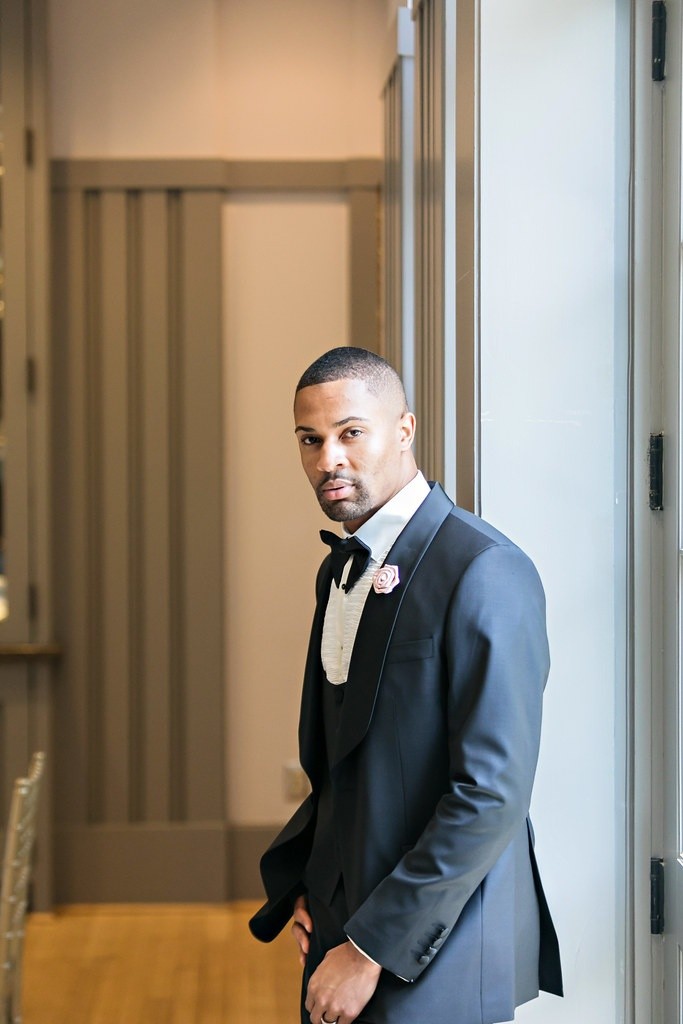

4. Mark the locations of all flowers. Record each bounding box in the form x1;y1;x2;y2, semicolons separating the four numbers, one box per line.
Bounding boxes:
371;563;401;594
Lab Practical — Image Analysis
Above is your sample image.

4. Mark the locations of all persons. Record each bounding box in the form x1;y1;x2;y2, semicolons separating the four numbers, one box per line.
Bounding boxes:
248;347;566;1024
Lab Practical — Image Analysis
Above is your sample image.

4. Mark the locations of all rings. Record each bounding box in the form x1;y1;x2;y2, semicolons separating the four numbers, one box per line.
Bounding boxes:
321;1017;336;1024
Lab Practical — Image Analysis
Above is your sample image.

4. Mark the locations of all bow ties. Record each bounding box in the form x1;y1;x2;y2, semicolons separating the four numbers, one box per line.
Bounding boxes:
319;527;370;595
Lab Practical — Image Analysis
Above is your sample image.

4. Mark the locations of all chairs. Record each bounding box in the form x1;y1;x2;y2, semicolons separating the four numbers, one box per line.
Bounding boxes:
0;749;47;1024
